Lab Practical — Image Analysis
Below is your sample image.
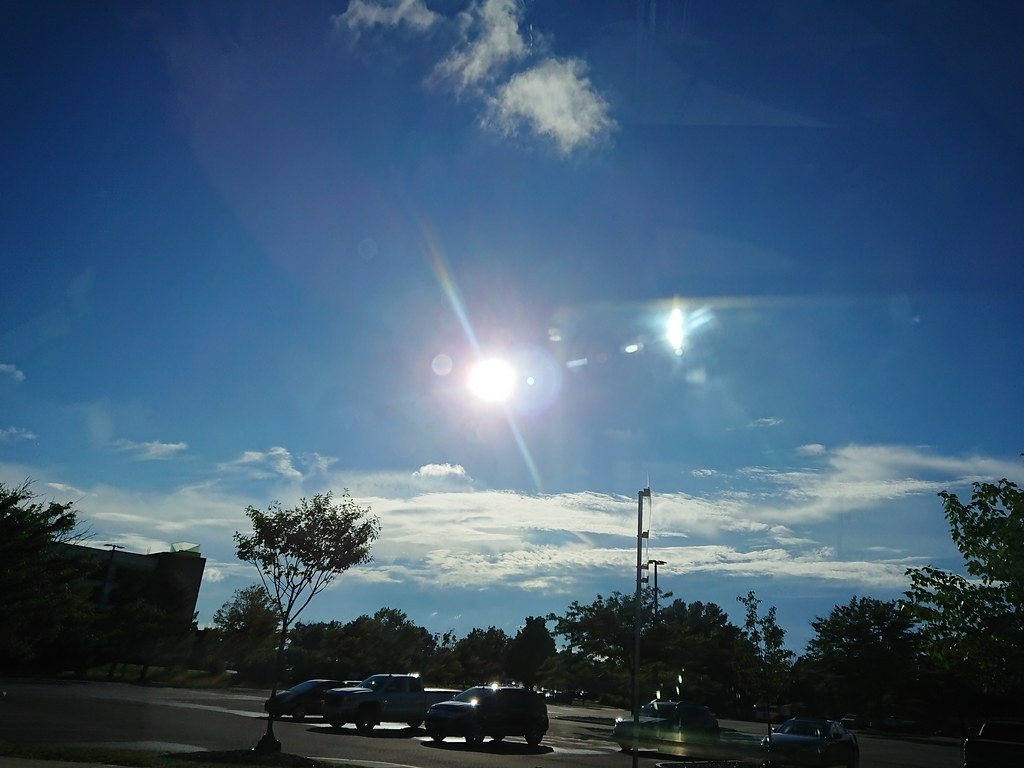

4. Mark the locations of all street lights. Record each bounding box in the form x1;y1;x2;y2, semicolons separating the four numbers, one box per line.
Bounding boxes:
632;489;651;768
97;544;125;587
648;560;666;613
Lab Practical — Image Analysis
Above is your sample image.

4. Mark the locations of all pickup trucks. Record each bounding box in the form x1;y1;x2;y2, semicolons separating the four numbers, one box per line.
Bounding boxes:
321;673;463;735
964;720;1024;768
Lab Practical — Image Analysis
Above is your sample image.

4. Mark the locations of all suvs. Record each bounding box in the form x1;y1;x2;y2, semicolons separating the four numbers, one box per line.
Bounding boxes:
610;698;721;753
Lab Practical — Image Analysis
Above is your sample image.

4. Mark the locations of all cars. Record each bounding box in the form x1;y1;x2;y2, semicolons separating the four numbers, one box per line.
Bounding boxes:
265;679;347;719
425;686;549;745
757;705;779;719
841;714;865;729
759;716;859;768
